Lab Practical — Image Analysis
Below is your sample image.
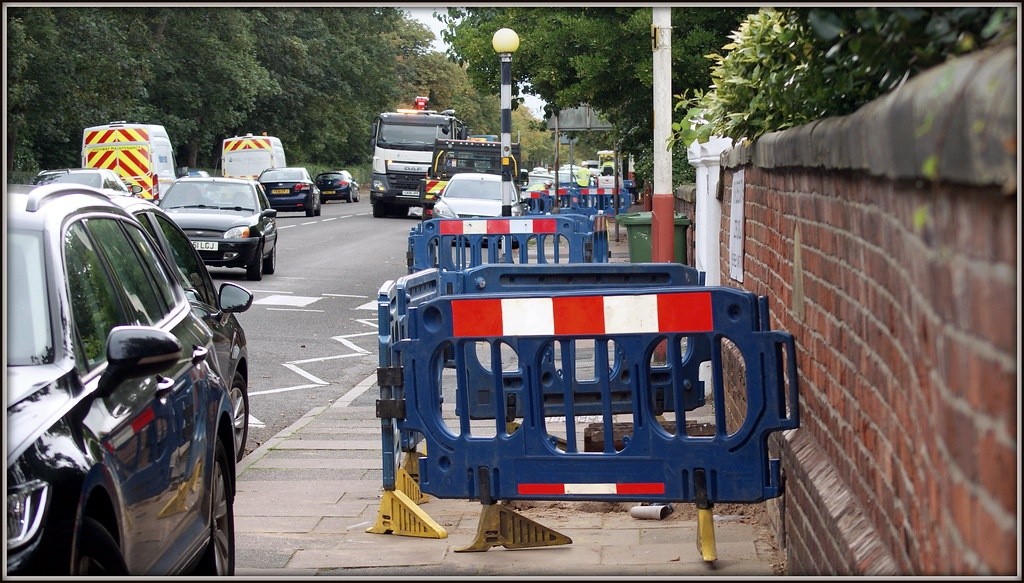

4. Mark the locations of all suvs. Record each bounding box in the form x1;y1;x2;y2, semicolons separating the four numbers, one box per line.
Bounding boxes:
7;181;235;575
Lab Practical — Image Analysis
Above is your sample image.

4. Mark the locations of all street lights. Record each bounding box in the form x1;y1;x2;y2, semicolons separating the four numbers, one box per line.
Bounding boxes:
492;26;519;177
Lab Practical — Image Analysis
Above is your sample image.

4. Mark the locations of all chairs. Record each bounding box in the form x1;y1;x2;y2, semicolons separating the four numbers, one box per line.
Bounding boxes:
231;192;252;208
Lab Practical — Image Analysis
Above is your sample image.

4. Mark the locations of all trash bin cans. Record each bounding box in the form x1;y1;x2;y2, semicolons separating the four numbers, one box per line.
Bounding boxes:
615;210;691;263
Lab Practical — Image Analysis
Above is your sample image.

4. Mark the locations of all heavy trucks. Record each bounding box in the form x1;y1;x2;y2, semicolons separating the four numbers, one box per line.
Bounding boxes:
370;96;468;218
419;138;528;220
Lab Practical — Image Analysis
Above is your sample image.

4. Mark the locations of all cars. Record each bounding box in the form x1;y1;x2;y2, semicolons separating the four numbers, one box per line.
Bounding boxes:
519;150;637;211
315;169;361;203
28;167;142;197
101;191;253;464
152;175;279;281
432;173;525;249
258;166;321;217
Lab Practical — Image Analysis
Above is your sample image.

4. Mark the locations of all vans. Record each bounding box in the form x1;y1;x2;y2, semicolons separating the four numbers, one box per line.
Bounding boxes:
221;131;286;182
80;119;180;209
